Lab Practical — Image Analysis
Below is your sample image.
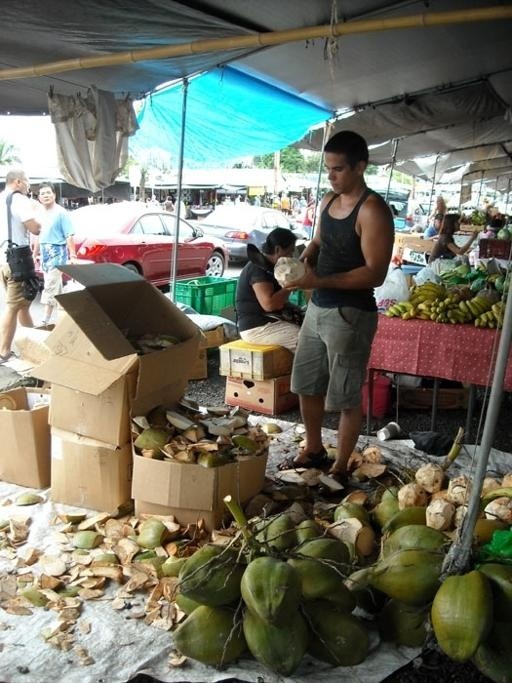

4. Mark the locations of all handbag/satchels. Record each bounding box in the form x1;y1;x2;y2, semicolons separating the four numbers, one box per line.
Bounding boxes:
6;243;37;283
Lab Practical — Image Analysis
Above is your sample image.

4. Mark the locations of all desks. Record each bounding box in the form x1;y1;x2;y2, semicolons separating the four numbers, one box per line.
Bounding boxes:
364;310;512;436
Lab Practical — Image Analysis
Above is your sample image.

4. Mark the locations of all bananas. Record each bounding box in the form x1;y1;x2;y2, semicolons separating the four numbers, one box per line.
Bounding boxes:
384;272;511;328
471;212;487;226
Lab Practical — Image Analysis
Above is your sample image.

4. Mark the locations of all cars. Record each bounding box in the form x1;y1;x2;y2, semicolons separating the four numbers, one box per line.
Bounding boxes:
192;192;475;269
30;202;231;296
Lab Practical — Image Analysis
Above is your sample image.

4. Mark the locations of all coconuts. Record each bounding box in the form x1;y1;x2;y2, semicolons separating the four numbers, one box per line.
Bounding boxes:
270;255;307;287
170;446;511;683
497;229;511;240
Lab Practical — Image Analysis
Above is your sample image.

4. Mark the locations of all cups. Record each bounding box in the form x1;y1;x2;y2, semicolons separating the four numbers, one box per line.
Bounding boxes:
377;421;403;440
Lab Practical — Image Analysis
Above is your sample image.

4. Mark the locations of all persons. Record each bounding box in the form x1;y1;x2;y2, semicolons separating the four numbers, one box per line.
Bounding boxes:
423;213;444;241
30;181;76;331
428;214;479;266
236;227;302;357
225;191;308;219
276;130;395;485
428;195;447;221
146;195;174;213
0;168;42;364
484;213;505;234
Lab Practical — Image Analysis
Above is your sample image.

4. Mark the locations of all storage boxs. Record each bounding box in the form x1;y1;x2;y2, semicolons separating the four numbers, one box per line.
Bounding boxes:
360;373;392;419
218;338;299;416
394;385;471;410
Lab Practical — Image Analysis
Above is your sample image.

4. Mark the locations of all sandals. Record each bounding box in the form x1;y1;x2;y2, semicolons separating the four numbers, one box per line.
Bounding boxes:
277;447;329;472
